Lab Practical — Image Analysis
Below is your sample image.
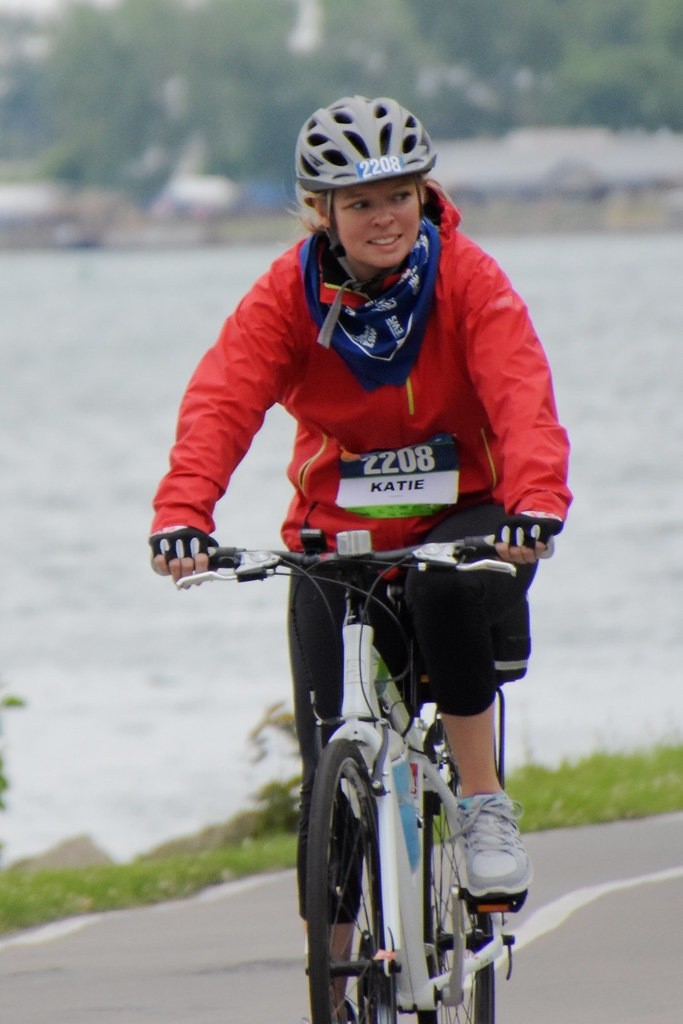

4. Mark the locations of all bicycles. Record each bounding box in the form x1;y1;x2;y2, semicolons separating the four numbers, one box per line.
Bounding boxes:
174;524;541;1024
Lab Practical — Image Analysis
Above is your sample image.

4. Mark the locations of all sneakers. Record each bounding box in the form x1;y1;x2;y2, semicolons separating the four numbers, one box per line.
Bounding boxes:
302;996;359;1024
449;791;531;900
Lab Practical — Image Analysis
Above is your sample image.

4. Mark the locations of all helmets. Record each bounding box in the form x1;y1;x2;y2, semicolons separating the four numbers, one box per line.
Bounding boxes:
294;95;436;191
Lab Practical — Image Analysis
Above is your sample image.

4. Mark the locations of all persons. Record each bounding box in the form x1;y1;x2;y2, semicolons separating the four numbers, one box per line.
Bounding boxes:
149;97;572;1024
